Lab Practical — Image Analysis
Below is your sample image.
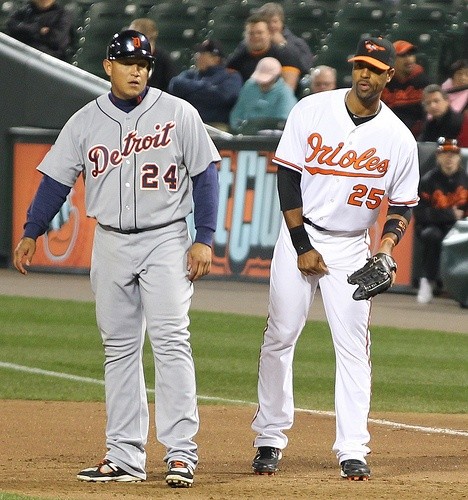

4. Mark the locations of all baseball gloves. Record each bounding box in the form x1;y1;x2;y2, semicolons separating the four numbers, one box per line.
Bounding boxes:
347;253;397;300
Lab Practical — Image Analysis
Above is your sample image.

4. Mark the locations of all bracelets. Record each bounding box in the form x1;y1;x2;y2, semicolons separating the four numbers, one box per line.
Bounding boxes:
276;41;288;50
380;237;395;247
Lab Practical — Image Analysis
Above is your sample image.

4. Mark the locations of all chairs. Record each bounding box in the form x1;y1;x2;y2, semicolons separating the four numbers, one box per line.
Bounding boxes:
0;0;468;99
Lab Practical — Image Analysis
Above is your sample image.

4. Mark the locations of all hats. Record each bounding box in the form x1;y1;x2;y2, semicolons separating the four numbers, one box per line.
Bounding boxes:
251;57;281;84
435;136;460;153
393;39;415;56
190;38;225;59
348;36;397;70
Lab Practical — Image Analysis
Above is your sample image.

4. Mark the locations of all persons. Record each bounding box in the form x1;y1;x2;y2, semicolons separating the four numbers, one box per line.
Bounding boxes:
412;136;468;304
250;35;420;482
380;40;430;109
4;0;72;58
426;60;468;120
168;37;243;124
128;18;175;93
309;65;337;94
410;84;463;143
226;2;314;84
12;29;222;487
230;57;298;134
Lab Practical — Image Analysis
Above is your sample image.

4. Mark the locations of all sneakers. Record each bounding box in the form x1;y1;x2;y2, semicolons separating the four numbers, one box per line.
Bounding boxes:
164;461;194;488
75;459;143;483
340;460;371;481
252;447;282;476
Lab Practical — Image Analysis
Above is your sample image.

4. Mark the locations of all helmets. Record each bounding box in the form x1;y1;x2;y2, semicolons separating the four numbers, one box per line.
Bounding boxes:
107;30;156;72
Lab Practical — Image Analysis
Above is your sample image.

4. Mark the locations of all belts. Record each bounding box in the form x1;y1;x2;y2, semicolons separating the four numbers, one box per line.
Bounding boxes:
97;217;186;235
302;215;329;231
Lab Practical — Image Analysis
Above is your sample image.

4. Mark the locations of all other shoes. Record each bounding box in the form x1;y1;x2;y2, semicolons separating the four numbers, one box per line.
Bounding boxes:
416;277;437;303
